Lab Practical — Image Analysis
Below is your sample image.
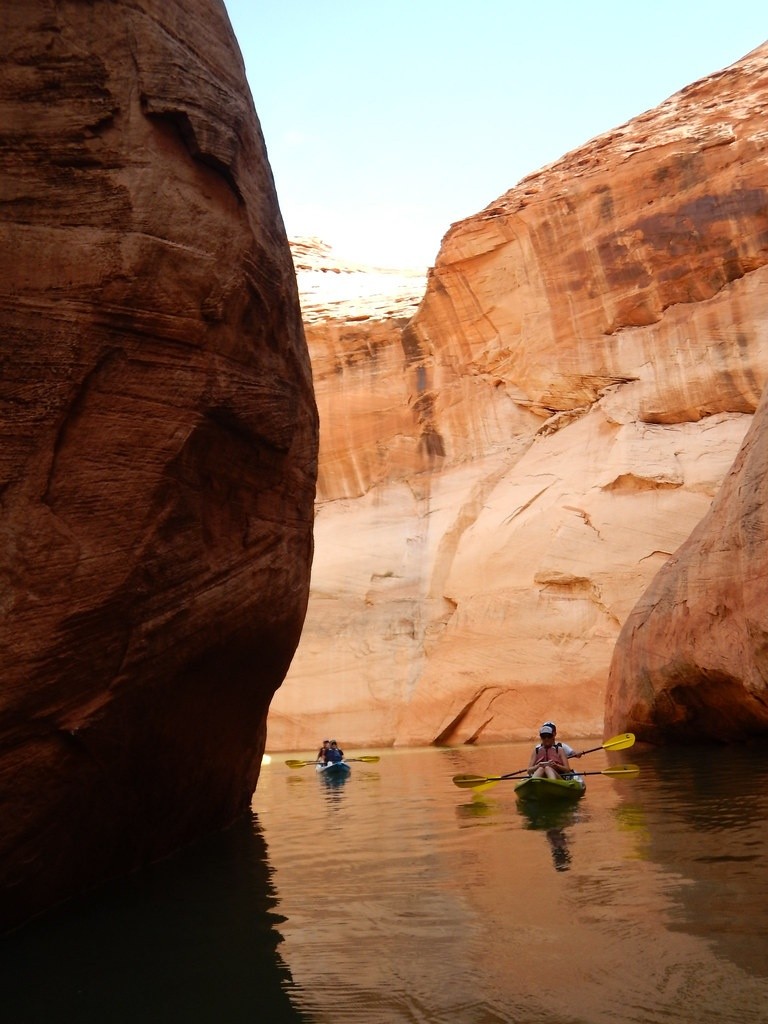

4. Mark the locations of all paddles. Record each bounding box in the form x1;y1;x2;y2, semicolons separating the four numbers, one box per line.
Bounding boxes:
284;756;381;769
452;763;640;789
472;733;635;792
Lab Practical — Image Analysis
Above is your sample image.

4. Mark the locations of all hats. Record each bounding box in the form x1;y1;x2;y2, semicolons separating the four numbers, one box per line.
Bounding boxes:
539;726;553;733
323;740;328;744
544;722;556;729
330;739;336;745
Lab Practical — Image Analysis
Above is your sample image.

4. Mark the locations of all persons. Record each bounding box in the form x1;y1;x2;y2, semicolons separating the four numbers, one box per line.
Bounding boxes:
315;739;346;768
527;722;582;781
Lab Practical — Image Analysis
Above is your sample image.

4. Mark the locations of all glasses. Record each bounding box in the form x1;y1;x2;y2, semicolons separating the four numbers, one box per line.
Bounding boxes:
541;734;553;739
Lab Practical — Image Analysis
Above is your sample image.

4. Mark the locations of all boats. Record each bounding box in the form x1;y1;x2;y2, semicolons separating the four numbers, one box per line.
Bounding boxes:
314;760;352;775
512;771;588;803
